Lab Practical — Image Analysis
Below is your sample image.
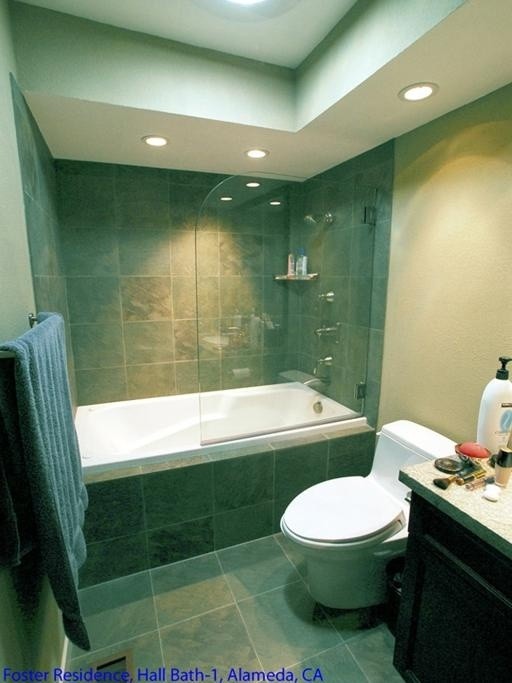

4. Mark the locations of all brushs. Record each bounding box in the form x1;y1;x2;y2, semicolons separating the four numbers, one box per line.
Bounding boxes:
434;467;486;489
432;466;473;489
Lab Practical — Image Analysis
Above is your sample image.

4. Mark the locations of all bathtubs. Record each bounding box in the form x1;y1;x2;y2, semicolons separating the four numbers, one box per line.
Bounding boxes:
73;381;369;474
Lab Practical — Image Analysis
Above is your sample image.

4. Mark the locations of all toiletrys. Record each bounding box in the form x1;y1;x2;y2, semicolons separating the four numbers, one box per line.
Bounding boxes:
494;447;512;486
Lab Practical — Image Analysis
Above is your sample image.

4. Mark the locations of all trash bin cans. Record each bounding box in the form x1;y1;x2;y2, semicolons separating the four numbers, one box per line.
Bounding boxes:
385;553;408;636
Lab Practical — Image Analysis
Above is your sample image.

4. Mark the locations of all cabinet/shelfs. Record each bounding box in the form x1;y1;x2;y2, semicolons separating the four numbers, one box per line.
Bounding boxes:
393;492;512;683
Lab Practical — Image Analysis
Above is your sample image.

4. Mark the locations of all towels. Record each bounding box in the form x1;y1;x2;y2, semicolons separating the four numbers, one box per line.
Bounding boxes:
0;315;91;651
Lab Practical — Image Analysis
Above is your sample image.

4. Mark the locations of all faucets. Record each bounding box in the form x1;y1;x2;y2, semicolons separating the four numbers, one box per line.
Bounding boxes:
301;377;331;390
228;326;241;334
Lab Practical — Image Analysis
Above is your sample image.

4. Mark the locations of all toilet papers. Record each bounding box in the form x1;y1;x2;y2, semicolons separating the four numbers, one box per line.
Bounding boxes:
230;367;251;379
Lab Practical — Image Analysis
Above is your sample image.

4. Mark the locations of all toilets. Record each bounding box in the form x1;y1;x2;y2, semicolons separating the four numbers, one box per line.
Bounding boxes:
278;418;458;609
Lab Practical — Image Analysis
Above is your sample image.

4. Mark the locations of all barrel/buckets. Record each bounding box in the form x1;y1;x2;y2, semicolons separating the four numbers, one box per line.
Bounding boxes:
202;334;229;354
